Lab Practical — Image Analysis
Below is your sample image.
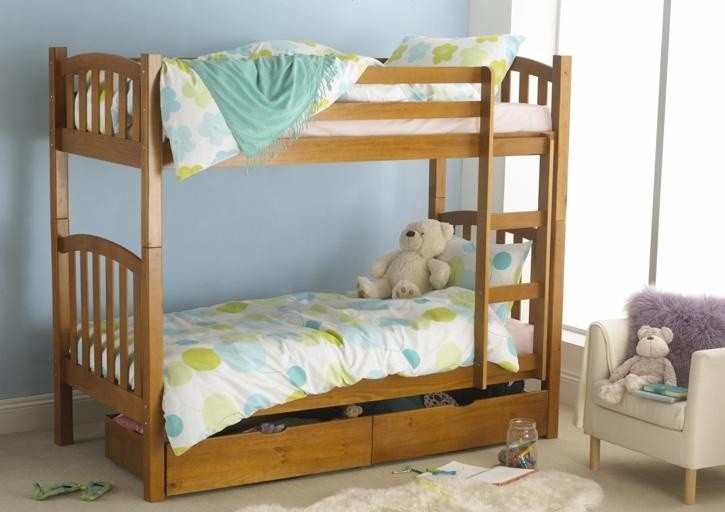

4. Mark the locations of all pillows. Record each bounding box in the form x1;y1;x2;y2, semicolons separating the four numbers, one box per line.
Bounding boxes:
380;32;526;105
431;236;534;315
630;286;724;387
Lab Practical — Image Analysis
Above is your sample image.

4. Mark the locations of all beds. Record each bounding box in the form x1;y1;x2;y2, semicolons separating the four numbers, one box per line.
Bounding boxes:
46;39;571;504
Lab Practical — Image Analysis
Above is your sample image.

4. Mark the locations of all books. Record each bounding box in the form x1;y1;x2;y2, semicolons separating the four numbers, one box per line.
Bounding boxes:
632;389;684;403
643;382;688;398
416;460;537;485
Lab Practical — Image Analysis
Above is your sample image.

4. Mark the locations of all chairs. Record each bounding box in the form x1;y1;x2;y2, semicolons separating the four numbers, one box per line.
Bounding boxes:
572;319;725;506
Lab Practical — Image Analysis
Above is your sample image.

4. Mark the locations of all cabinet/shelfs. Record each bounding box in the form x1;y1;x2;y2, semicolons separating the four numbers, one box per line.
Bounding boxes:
100;384;548;495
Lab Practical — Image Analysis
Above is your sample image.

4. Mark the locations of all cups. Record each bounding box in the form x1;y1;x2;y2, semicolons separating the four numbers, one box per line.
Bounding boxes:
506;418;539;470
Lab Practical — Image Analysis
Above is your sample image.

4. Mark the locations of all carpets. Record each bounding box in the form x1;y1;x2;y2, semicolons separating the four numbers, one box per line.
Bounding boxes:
241;468;605;512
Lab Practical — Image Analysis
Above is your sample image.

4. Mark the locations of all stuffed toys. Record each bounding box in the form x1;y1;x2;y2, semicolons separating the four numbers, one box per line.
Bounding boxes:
594;324;676;405
357;219;455;300
